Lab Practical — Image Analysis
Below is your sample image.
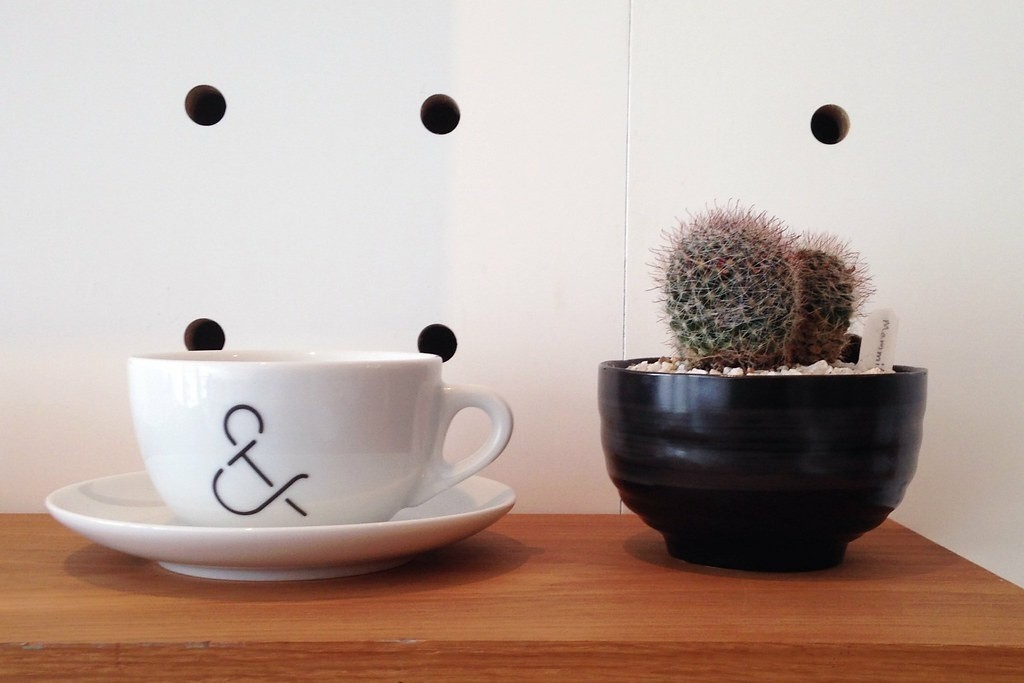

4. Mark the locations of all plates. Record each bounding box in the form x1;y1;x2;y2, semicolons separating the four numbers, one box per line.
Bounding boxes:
45;471;517;581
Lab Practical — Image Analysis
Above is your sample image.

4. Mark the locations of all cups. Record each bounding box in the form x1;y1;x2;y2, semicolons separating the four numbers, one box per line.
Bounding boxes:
128;349;514;527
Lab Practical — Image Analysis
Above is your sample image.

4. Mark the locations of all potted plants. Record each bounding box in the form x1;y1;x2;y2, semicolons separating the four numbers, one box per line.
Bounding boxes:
598;198;929;573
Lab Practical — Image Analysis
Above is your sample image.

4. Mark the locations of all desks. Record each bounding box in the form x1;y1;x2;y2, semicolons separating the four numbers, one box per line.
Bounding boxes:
0;513;1024;683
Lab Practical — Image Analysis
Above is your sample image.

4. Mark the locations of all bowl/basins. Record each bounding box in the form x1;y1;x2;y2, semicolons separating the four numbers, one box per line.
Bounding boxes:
598;358;928;573
